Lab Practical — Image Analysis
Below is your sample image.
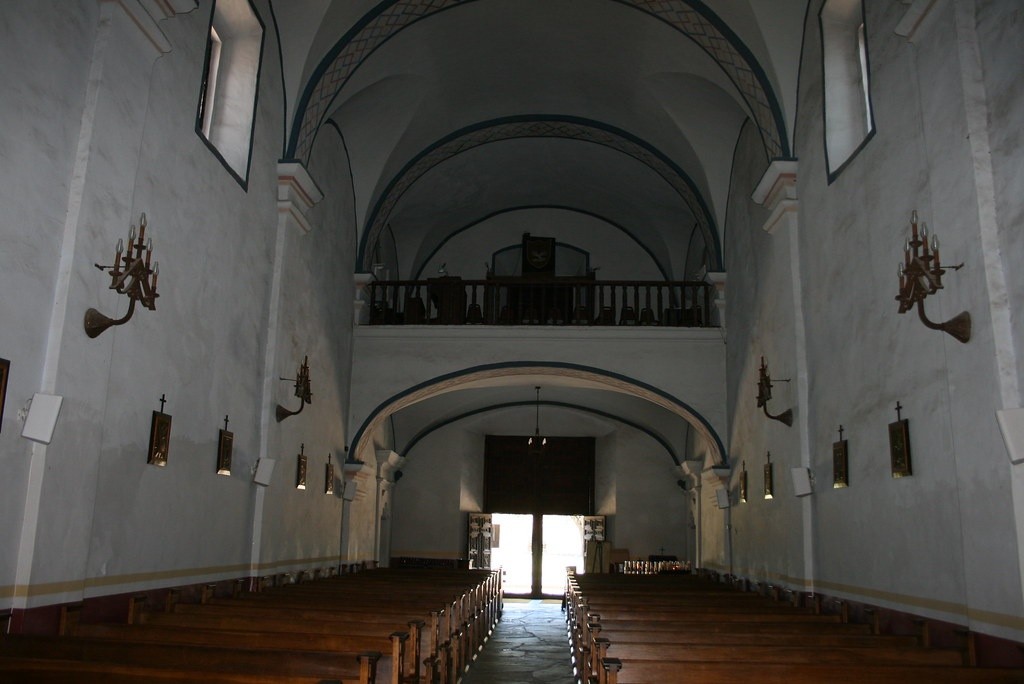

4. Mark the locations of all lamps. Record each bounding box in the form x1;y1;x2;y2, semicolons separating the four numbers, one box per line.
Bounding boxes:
276;355;313;423
895;210;971;343
528;386;552;452
84;212;161;338
756;356;792;427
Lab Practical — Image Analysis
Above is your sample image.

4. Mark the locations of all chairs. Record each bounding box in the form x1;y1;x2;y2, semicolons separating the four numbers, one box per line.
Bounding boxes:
466;304;702;325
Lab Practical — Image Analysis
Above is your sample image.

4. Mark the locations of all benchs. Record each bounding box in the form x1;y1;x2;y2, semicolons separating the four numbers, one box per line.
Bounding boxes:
565;573;1024;684
1;568;503;684
